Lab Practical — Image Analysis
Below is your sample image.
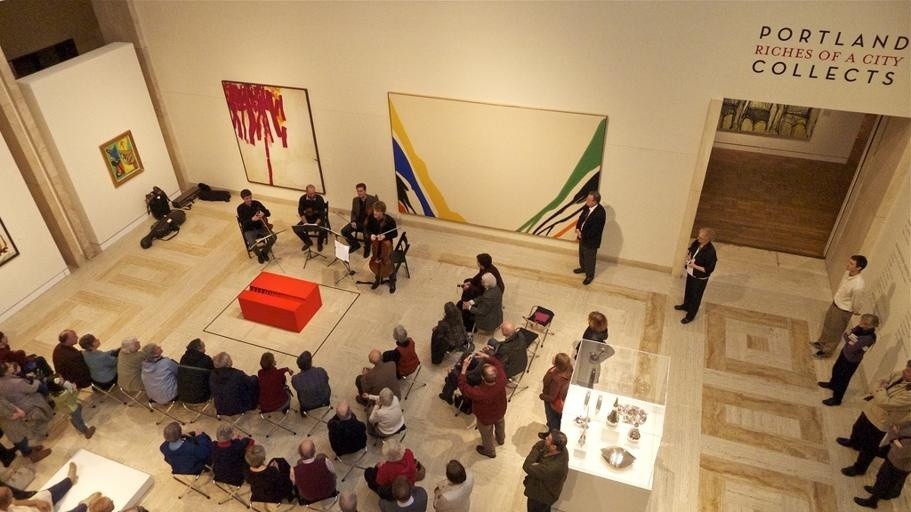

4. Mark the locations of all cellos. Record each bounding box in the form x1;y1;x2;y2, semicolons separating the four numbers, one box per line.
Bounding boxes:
369;216;395;277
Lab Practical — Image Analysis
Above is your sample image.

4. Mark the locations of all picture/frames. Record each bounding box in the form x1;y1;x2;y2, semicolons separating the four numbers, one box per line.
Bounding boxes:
100;130;144;188
0;218;20;268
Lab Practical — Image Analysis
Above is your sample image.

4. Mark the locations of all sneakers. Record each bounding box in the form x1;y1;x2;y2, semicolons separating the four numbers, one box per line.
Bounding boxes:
836;437;858;450
812;351;833;359
841;465;868;476
495;430;504;445
476;444;496;458
67;462;78;485
537;431;549;440
439;393;453;405
77;492;103;508
444;377;458;392
809;340;826;349
84;426;96;439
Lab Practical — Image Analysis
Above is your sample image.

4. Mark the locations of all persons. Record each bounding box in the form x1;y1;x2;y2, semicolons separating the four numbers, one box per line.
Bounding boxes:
836;360;911;477
51;329;331;411
717;98;814;138
0;462;114;512
341;182;376;258
366;200;397;294
573;190;607;285
157;421;338;501
236;189;278;264
673;227;718;324
293;184;325;252
809;254;868;359
817;314;880;406
853;413;911;509
524;312;608;512
432;253;529;457
0;330;96;467
327;325;474;512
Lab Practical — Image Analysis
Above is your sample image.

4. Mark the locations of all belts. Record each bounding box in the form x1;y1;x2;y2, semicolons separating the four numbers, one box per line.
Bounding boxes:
833;300;852;314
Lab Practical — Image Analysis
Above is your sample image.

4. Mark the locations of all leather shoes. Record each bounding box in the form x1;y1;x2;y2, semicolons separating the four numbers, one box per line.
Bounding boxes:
574;268;586;274
317;242;323;252
371;279;380;289
258;252;269;264
864;485;876;494
583;274;594;285
349;244;361;254
390;283;395;293
818;381;836;390
822;397;841;406
21;490;37;498
675;305;687;311
681;318;693;324
853;497;877;509
302;242;313;251
2;447;17;467
363;245;371;258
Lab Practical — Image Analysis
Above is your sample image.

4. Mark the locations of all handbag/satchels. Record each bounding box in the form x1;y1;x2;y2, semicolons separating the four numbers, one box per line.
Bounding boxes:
533;311;549;322
453;394;473;415
416;459;426;482
550;398;564;414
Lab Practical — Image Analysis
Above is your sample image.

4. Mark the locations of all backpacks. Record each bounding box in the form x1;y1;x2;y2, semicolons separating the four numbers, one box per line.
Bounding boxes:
873;444;891;460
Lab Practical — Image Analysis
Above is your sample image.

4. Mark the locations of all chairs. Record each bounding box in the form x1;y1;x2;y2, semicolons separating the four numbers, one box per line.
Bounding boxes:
236;201;410;281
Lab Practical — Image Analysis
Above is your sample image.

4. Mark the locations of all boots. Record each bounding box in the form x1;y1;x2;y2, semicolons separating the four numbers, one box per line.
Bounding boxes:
22;445;53;463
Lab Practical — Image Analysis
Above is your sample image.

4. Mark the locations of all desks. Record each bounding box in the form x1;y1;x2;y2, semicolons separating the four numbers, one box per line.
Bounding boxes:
34;450;155;512
238;273;322;334
